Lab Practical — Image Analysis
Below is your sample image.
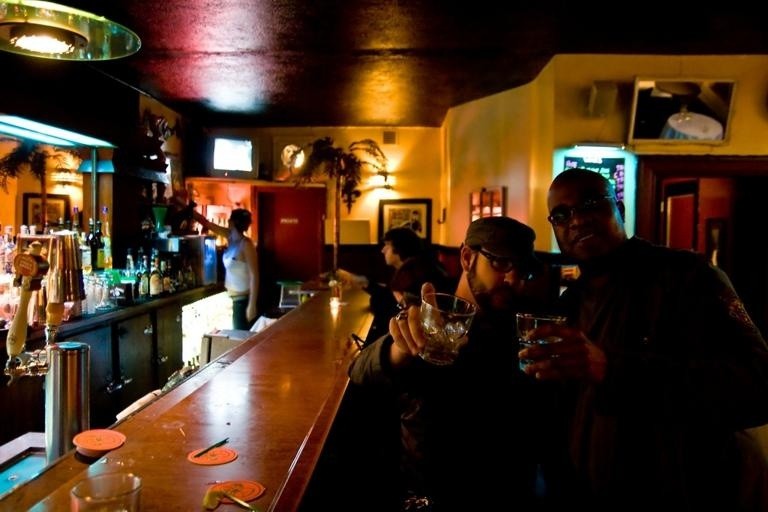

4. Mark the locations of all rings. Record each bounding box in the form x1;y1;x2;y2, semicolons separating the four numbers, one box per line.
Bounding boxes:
394;312;410;322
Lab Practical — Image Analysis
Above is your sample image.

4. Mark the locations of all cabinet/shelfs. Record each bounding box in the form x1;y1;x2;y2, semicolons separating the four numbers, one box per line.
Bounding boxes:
154;299;179;373
64;319;115;409
115;309;154;394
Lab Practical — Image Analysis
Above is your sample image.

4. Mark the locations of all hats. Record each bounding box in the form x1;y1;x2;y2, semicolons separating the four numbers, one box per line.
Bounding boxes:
466;216;536;261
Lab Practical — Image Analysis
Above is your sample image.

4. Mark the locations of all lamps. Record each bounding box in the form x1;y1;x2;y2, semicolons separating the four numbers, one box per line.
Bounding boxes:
0;0;142;61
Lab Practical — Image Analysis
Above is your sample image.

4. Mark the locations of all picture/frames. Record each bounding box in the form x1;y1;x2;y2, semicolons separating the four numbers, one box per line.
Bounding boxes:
22;192;71;235
378;198;432;245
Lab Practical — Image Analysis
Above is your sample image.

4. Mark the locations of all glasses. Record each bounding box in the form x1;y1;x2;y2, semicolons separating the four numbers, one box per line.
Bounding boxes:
478;249;533;280
548;195;615;225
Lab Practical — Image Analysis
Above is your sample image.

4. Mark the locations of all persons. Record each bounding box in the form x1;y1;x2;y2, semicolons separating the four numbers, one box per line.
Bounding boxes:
348;217;562;511
335;229;455;315
164;189;197;234
518;170;768;512
204;209;260;330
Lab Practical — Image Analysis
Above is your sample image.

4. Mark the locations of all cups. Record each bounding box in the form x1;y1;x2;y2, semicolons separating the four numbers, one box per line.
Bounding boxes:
418;292;477;366
70;472;141;512
515;312;567;373
328;280;343;298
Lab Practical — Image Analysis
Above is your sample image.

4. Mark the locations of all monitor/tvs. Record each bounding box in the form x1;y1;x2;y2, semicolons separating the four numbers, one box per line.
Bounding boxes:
206;133;259;179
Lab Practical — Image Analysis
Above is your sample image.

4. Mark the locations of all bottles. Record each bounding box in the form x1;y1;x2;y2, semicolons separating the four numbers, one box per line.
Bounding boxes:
0;206;176;327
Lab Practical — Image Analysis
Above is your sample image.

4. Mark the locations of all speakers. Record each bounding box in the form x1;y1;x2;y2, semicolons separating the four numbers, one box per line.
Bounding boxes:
586;80;618;118
